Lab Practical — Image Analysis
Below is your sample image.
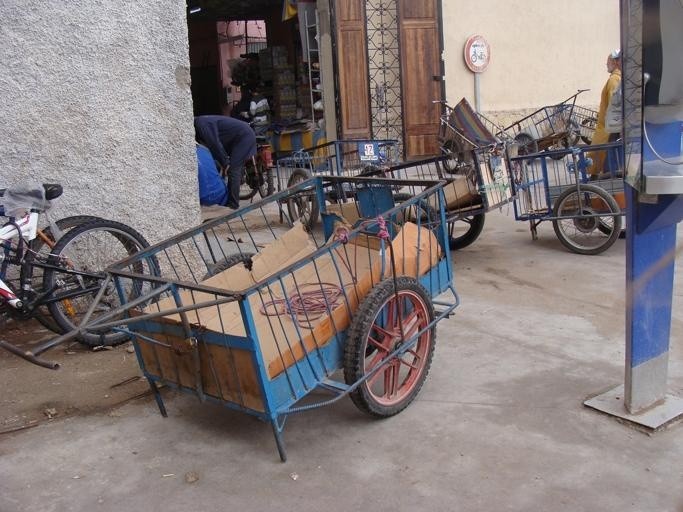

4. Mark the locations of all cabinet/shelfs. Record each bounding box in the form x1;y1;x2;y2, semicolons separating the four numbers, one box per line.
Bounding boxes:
302;10;325;130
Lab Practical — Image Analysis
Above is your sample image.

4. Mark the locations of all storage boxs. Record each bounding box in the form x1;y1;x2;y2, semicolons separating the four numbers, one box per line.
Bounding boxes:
257;46;318;121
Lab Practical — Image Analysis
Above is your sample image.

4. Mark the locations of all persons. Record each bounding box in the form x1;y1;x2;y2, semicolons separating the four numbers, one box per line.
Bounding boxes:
240;85;270;136
577;48;622;178
605;50;624;170
196;141;229;208
193;114;256;210
